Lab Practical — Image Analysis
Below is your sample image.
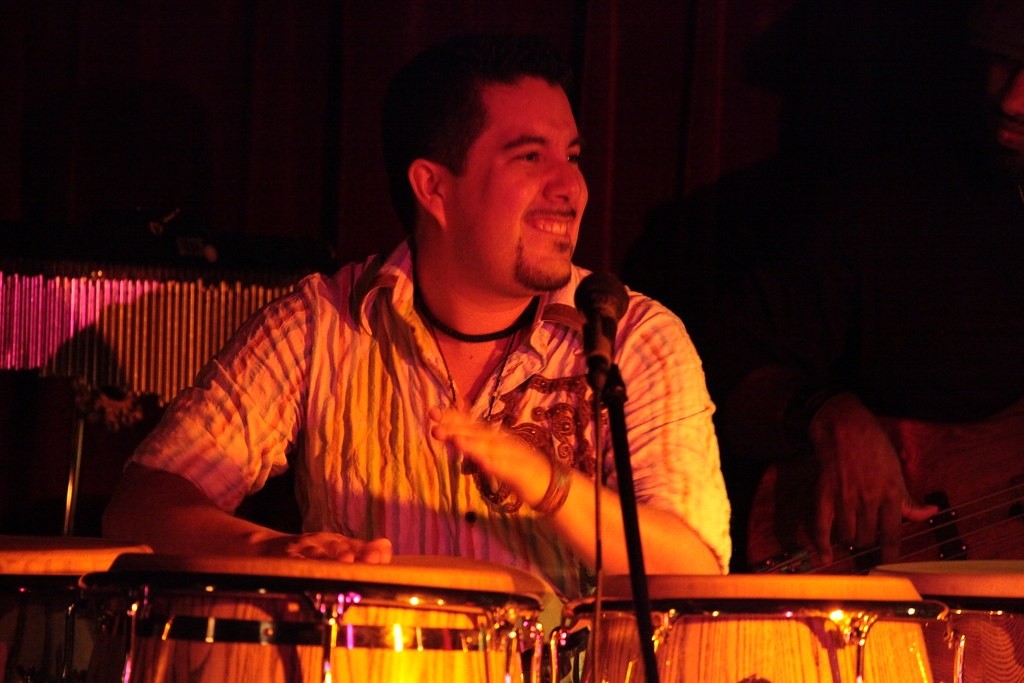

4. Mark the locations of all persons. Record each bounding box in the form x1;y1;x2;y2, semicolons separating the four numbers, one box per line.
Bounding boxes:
683;1;1023;615
101;34;733;597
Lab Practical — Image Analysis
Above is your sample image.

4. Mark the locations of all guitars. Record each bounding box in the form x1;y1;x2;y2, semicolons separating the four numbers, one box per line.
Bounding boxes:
744;409;1024;574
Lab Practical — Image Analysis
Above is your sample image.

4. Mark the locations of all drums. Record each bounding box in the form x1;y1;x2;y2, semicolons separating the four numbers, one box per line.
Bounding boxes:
88;553;568;683
0;542;153;681
576;573;932;682
869;559;1024;682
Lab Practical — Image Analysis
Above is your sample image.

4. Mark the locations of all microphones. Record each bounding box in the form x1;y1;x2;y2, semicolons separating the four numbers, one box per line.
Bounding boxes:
575;272;631;390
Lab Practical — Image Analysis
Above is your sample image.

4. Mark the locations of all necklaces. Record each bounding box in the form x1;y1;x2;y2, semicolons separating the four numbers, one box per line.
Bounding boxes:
428;321;515;474
424;301;527;341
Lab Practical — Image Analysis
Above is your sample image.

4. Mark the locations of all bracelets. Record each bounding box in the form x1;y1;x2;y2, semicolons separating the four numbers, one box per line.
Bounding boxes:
535;447;572;513
